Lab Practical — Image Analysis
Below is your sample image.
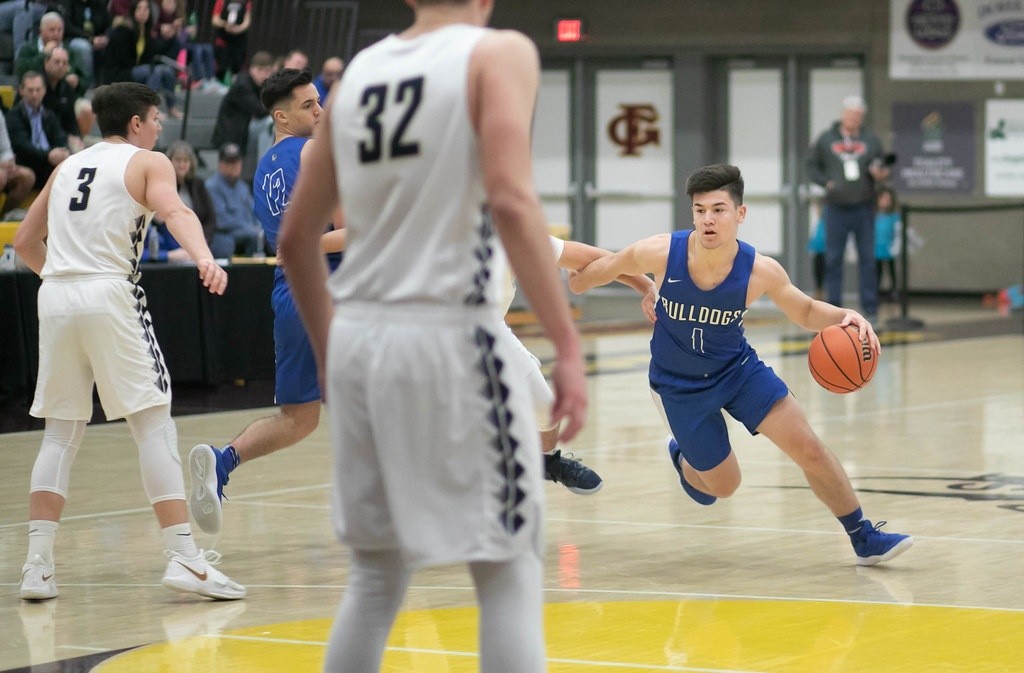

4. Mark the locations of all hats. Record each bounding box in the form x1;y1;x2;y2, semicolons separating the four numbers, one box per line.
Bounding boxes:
218;141;241;164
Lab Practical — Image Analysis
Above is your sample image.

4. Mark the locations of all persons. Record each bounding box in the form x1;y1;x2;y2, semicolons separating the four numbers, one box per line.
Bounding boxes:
187;68;343;535
568;164;914;567
0;0;343;263
12;85;245;602
278;227;658;494
806;187;903;301
804;96;884;333
276;0;586;673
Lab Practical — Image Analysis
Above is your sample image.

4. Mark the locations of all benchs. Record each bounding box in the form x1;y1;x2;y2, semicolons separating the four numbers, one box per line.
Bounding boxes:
0;33;229;155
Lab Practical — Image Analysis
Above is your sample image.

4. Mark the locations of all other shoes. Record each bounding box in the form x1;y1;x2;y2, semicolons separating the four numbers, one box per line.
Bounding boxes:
198;77;231;96
3;207;30;222
864;314;879;331
167;106;186;120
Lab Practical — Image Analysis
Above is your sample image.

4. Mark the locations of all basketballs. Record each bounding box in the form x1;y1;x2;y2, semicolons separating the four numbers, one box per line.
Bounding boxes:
808;323;878;394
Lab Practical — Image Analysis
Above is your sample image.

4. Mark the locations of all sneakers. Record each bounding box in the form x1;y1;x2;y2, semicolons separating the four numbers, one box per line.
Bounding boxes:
17;555;60;600
188;444;230;534
853;519;914;566
162;601;246;646
160;548;246;599
543;450;604;495
19;600;57;643
664;435;717;506
